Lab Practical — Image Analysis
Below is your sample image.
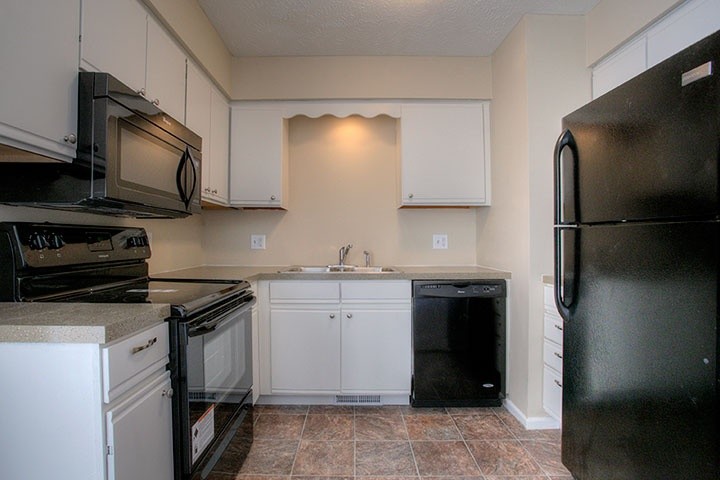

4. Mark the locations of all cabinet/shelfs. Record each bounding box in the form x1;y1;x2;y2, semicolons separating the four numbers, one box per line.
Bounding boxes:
396;101;492;208
269;281;412;394
0;0;81;164
588;0;720;101
249;283;260;403
0;321;174;480
186;60;230;208
81;0;187;126
230;103;288;211
542;282;564;422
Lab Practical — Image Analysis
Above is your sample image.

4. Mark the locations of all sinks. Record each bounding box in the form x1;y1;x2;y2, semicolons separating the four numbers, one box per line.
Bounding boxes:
286;265;342;273
342;265;395;273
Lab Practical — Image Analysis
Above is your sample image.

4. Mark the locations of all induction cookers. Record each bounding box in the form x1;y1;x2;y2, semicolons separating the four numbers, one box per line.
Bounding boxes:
0;221;255;318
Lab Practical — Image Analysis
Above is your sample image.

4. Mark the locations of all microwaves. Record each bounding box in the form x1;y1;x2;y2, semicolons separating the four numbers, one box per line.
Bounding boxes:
0;71;203;220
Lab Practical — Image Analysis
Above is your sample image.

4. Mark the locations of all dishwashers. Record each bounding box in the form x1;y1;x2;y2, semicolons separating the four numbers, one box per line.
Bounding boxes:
411;279;507;408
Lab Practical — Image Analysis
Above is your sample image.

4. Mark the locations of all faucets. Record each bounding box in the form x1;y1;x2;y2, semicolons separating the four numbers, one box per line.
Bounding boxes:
362;250;371;267
338;242;354;265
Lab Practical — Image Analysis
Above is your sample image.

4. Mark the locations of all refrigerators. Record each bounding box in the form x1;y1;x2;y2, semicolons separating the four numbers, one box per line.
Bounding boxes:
553;31;720;480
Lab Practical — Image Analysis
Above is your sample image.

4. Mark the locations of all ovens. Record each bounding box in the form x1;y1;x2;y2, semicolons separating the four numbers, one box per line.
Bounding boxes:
169;289;255;480
